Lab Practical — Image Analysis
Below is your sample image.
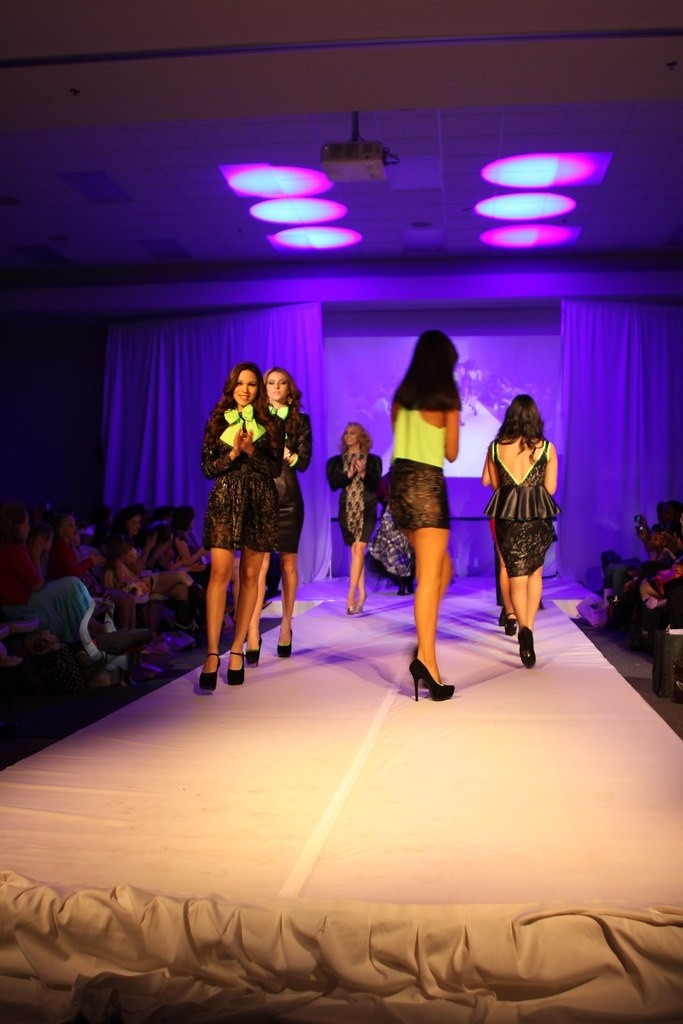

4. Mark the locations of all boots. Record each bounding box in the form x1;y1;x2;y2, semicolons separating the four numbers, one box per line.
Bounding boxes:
174;599;193;638
188;581;233;617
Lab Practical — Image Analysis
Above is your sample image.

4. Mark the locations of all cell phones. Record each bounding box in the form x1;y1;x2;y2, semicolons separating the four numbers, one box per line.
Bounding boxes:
635;515;643;521
637;526;644;530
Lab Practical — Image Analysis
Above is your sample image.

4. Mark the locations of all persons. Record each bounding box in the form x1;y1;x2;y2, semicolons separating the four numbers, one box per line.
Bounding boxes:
0;501;283;688
326;421;382;616
197;362;285;693
481;393;561;668
390;329;462;701
366;467;417;595
453;357;527;424
245;367;312;667
602;500;683;654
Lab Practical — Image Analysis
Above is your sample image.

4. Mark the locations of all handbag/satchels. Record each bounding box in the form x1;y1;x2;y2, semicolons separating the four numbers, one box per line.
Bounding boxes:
30;640;84;693
128;650;173;684
576;598;608;630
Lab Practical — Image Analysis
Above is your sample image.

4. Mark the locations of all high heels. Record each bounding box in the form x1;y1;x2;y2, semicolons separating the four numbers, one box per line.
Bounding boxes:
499;606;506;627
506;612;518;634
199;653;221;690
246;636;262;664
518;627;537;668
228;652;245;685
277;629;293;657
408;658;455;700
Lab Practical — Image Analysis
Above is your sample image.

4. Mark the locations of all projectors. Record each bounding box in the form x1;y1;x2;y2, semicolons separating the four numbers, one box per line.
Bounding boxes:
320;141;387;182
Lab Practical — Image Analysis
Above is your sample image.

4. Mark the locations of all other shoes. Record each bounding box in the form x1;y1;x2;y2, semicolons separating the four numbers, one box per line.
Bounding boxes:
0;654;22;667
605;622;626;631
407;581;414;593
607;595;636;610
346;600;355;614
356;600;364;614
398;583;405;595
